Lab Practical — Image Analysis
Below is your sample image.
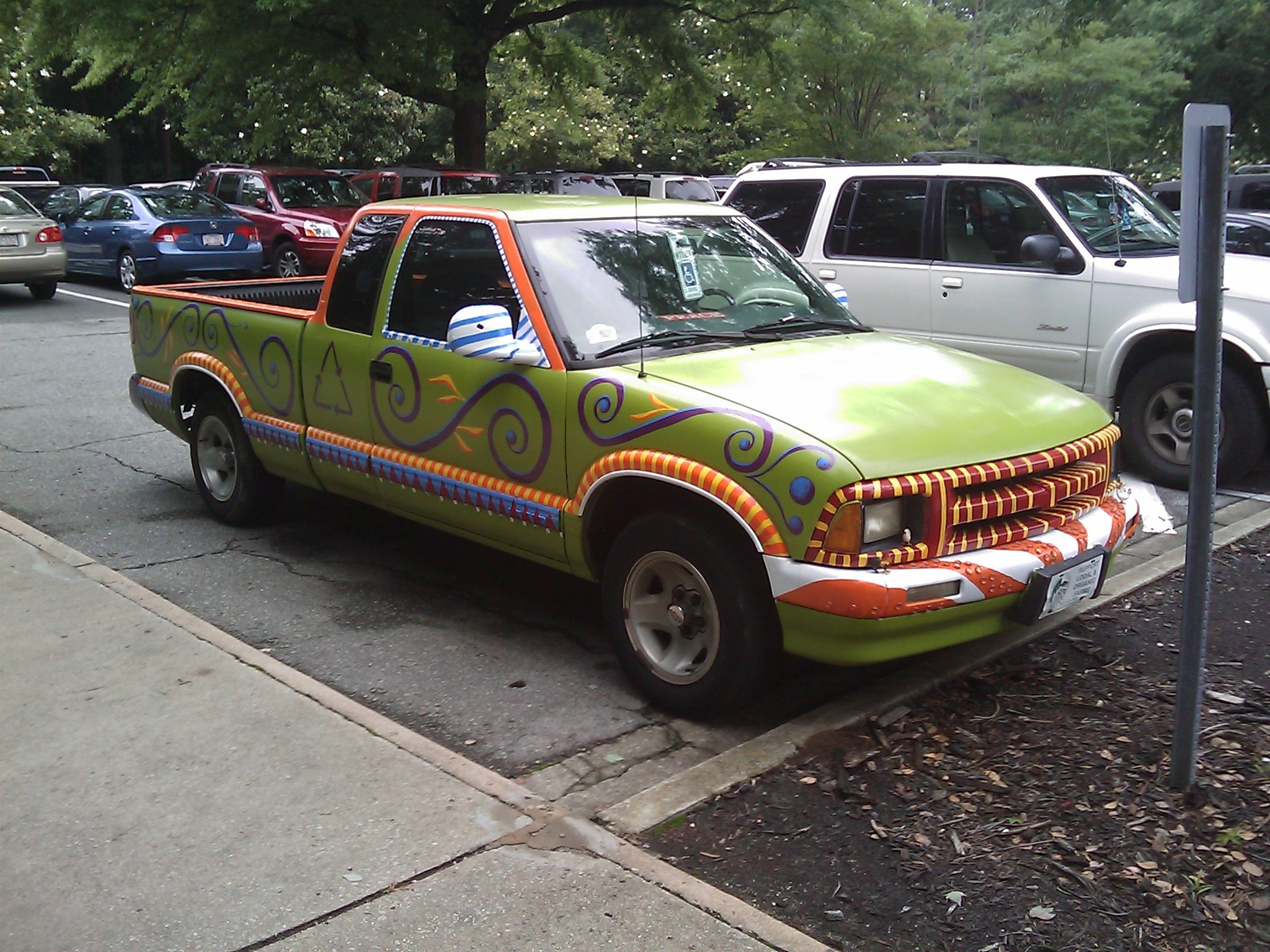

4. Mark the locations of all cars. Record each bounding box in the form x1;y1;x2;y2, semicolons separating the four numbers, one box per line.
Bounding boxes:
0;184;67;301
32;182;118;224
55;183;266;294
311;168;1270;254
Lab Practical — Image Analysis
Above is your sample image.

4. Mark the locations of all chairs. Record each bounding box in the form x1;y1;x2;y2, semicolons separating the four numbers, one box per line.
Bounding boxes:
1006;206;1043;263
945;204;998;265
842;213;875;257
884;208;919;258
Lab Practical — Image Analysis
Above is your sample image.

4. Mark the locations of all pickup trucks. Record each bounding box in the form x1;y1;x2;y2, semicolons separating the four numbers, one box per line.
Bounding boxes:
0;166;61;209
126;198;1145;728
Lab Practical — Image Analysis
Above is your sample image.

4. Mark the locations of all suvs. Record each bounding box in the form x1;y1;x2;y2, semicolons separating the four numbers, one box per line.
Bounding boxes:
191;161;374;279
697;153;1270;493
